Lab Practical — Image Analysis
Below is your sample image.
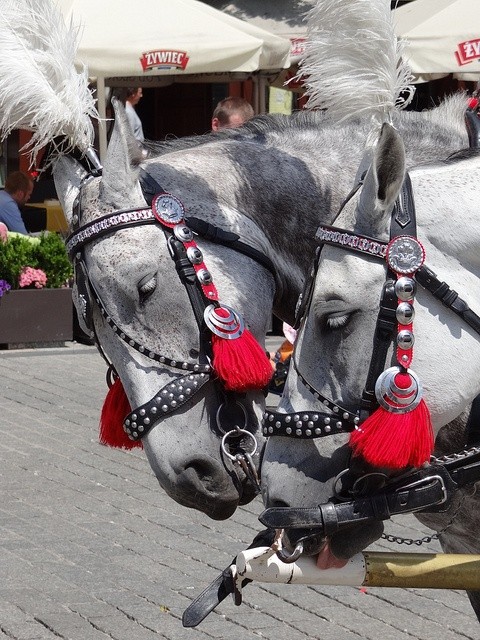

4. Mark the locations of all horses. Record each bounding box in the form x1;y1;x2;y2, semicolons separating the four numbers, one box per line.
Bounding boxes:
260;122;480;570
49;88;480;521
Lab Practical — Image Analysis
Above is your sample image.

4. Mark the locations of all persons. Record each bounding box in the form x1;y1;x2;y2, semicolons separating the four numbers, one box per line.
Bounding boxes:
211;96;255;132
0;170;33;235
119;86;144;146
